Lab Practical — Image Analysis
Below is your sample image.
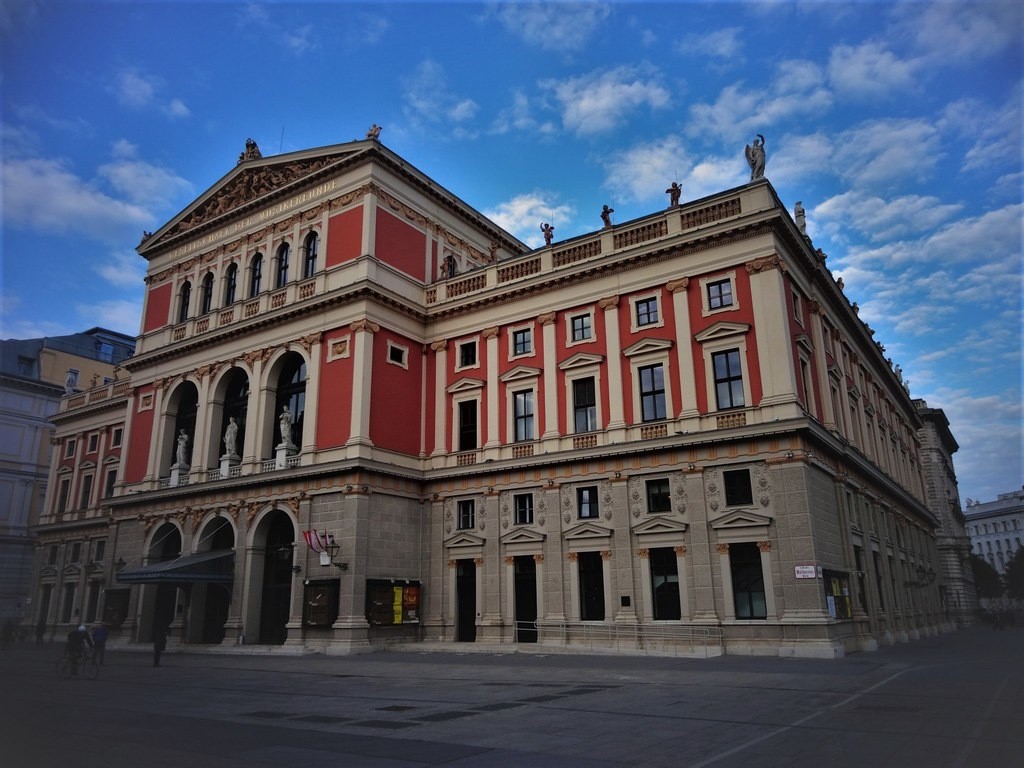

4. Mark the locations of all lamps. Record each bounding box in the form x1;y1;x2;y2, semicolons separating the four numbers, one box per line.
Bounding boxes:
113;557;127;572
325;539;349;572
82;559;106;587
278;546;301;573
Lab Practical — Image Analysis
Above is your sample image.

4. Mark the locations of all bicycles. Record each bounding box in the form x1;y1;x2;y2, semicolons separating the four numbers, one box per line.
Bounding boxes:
56;648;100;680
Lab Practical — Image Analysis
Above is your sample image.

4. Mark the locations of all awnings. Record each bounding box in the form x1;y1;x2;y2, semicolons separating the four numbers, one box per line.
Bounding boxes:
115;549;238;583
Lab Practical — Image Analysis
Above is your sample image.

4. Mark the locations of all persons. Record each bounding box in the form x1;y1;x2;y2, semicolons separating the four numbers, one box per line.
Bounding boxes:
600;205;614;227
175;429;190;465
440;258;455;278
888;358;893;368
160;156;344;242
368;124;377;137
112;365;122;381
794;200;806;235
992;603;1004;631
904;382;911;395
865;322;875;338
836;277;844;292
89;373;100;387
751;133;766;179
32;617;46;645
540;223;553;245
64;623;110;675
224;416;239;455
487;241;497;263
877;342;886;355
816;247;828;264
279;405;293;443
666;182;681;209
852;302;859;316
152;614;171;667
895;364;903;381
245;137;259;159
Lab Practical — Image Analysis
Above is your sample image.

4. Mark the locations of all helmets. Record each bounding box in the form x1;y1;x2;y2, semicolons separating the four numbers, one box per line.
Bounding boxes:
78;626;86;632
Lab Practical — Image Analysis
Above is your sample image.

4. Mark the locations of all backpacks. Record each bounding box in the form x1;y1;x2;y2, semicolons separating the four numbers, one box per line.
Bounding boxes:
67;632;80;645
